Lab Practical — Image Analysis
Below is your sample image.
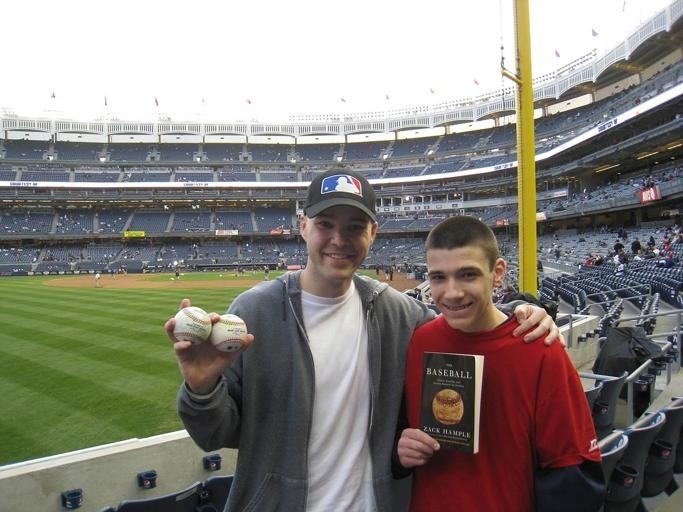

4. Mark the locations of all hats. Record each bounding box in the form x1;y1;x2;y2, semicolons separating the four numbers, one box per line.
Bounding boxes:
304;167;378;223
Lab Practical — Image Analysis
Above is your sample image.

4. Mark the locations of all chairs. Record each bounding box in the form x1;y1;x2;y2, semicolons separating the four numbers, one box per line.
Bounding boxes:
537;265;683;512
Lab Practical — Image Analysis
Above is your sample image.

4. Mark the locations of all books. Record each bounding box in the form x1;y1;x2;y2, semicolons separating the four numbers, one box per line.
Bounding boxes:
420;351;484;455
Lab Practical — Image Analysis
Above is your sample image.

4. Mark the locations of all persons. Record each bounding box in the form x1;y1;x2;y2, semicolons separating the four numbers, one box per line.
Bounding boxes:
165;167;567;512
390;216;606;512
0;61;682;315
340;71;344;80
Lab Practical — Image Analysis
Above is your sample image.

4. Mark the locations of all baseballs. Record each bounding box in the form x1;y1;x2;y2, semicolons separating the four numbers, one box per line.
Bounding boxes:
173;307;212;347
211;314;247;352
